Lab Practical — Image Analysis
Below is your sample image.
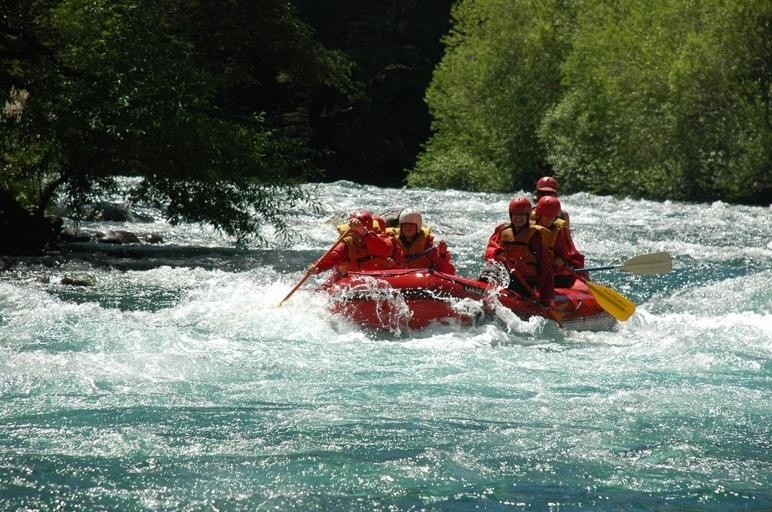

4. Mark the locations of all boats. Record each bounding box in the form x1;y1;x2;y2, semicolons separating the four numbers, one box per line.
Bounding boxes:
328;270;615;343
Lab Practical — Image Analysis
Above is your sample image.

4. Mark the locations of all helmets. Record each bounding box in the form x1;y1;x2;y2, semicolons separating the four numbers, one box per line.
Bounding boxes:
351;209;421;236
509;177;561;224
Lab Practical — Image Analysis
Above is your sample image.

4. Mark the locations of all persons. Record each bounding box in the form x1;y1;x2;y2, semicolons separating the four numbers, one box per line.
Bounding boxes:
532;174;585;269
529;195;576;287
394;207;456;275
305;208;398;274
484;195;557;308
313;214;402;293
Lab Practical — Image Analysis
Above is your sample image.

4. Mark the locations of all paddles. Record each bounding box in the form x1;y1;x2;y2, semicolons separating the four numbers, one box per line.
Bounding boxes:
575;251;672;274
563;264;636;321
